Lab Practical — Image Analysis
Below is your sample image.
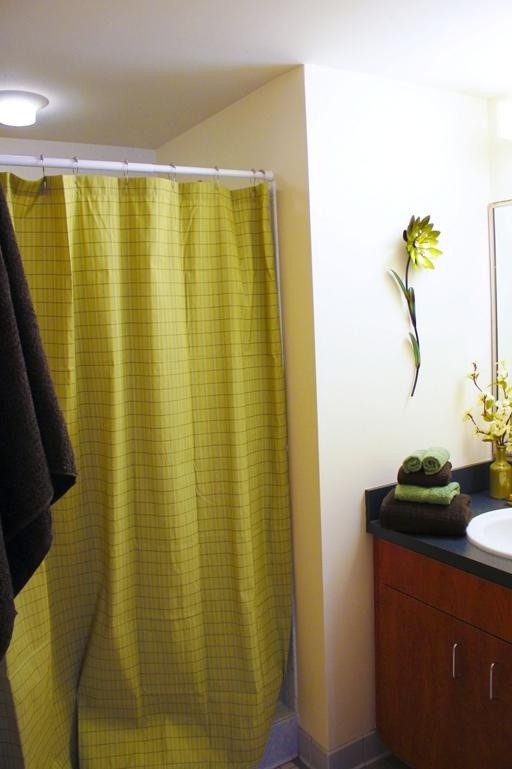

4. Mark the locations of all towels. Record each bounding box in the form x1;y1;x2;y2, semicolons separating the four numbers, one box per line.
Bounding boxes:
375;445;475;539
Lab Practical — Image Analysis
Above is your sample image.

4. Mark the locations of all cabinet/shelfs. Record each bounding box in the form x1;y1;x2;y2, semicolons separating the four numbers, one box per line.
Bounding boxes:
366;546;510;767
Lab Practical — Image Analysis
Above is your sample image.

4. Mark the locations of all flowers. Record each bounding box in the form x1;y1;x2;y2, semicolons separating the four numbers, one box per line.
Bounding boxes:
460;354;512;447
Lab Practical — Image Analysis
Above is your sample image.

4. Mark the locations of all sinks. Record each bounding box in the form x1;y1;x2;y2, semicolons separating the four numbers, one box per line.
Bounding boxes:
464;506;512;561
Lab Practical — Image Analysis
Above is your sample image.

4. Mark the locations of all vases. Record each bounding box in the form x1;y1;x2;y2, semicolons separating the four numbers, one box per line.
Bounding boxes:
490;450;512;500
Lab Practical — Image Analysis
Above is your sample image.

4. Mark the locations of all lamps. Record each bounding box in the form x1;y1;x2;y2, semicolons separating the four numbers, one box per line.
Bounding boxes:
0;89;50;127
